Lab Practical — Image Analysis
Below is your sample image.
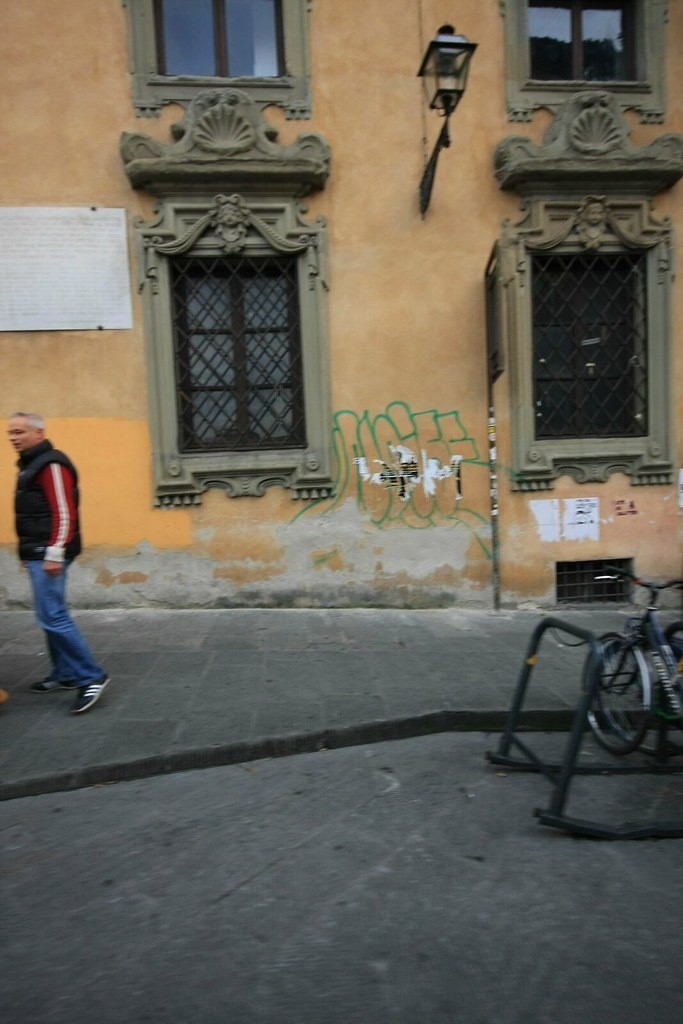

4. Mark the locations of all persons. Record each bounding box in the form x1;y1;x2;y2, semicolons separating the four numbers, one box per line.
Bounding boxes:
6;410;113;715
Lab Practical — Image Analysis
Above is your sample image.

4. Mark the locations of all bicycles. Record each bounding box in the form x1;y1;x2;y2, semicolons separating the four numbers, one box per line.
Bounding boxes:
580;563;683;758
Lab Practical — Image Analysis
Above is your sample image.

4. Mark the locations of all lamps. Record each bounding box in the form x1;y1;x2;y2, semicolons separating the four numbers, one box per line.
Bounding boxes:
415;22;478;217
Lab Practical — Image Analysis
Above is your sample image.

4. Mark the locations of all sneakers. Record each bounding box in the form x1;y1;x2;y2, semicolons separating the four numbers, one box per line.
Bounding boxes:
70;674;111;712
27;676;77;692
